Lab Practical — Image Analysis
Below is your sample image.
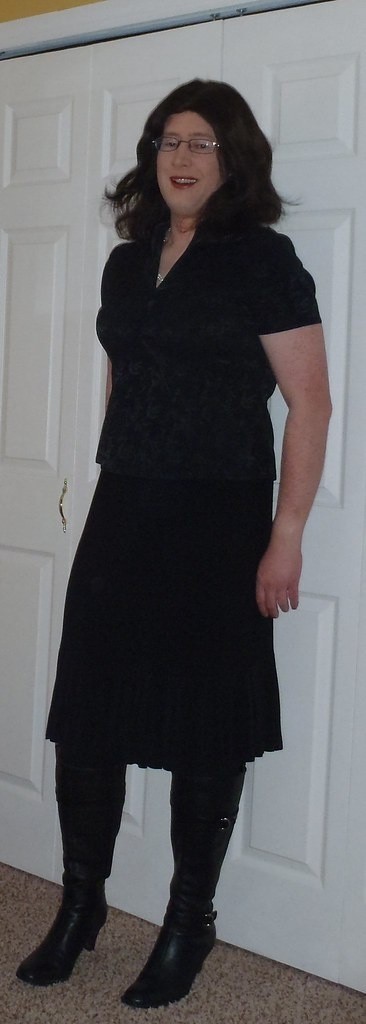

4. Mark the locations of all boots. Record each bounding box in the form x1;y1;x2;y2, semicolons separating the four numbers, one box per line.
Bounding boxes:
16;764;127;987
121;768;245;1008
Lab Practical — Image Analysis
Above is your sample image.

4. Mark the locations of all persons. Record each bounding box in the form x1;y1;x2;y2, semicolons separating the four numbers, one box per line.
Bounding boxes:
15;79;334;1008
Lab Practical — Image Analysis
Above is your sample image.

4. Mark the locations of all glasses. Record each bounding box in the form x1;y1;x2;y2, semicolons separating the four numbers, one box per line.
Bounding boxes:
152;135;224;154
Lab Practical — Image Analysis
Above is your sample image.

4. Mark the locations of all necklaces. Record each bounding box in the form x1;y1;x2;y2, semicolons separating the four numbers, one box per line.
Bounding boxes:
157;228;170;282
